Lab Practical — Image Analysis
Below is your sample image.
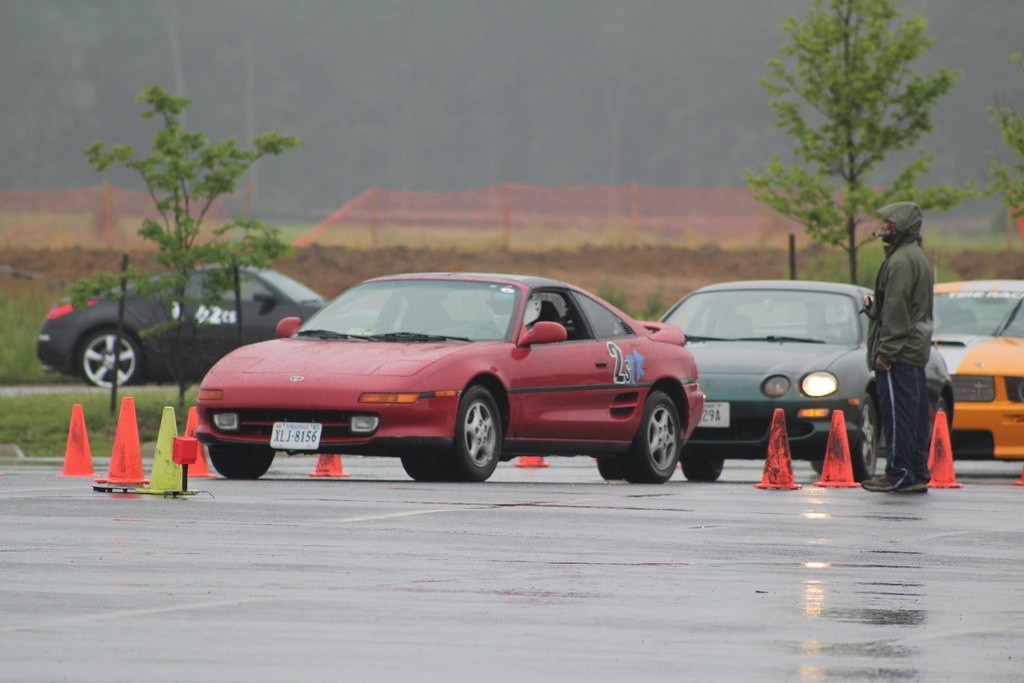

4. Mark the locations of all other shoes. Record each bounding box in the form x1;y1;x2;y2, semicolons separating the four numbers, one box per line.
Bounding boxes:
912;480;928;492
862;476;914;492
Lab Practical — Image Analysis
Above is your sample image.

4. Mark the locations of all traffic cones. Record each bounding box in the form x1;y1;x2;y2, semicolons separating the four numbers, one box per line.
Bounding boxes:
93;396;151;486
925;406;965;490
55;403;101;478
753;407;801;490
513;455;551;468
183;407;219;478
131;406;188;495
306;454;352;477
811;409;862;489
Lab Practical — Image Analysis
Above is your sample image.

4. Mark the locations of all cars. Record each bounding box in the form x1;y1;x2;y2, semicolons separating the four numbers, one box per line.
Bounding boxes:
926;275;1024;462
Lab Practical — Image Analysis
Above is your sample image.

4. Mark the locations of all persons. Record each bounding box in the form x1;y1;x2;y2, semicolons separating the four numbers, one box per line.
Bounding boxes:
804;299;852;342
479;290;541;336
864;202;934;493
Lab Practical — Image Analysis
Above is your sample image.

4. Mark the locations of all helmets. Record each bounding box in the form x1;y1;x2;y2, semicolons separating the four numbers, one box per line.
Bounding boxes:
490;287;542;335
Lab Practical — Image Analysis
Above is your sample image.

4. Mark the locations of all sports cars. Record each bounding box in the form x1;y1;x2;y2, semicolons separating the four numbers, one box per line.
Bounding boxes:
192;268;705;489
654;278;956;488
35;261;335;390
